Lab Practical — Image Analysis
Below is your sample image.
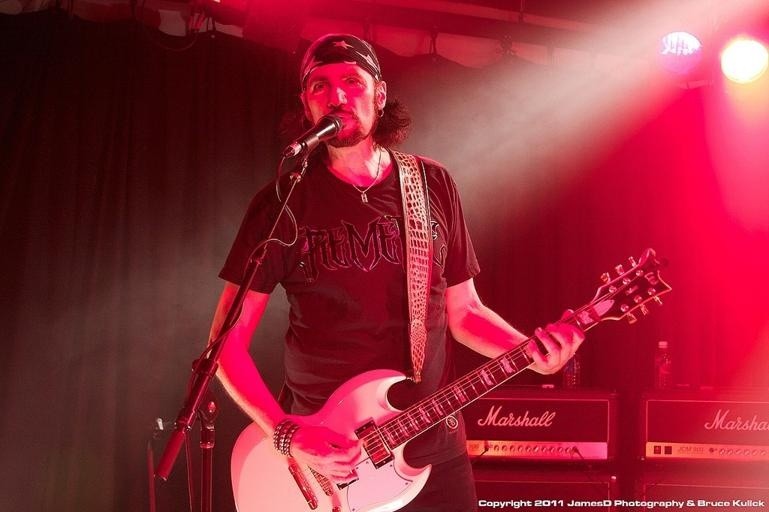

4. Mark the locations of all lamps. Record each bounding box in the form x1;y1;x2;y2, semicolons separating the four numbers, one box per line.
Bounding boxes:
659;15;705;78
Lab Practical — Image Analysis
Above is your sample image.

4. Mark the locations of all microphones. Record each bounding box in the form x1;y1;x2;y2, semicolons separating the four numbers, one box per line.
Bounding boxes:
283;114;342;158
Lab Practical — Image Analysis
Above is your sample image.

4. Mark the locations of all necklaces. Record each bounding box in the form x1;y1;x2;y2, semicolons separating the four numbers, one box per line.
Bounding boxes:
331;146;382;203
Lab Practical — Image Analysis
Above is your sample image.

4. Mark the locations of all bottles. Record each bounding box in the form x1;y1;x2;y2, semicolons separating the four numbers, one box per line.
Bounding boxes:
652;340;673;393
564;350;580;390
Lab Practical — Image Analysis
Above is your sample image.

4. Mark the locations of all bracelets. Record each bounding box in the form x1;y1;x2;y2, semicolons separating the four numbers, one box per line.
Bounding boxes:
273;416;297;457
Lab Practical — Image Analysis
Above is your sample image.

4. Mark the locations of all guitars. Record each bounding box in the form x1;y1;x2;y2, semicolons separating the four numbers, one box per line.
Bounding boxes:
230;248;672;512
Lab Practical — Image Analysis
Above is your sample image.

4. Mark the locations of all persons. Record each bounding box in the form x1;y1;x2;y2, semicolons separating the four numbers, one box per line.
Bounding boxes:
209;32;588;510
